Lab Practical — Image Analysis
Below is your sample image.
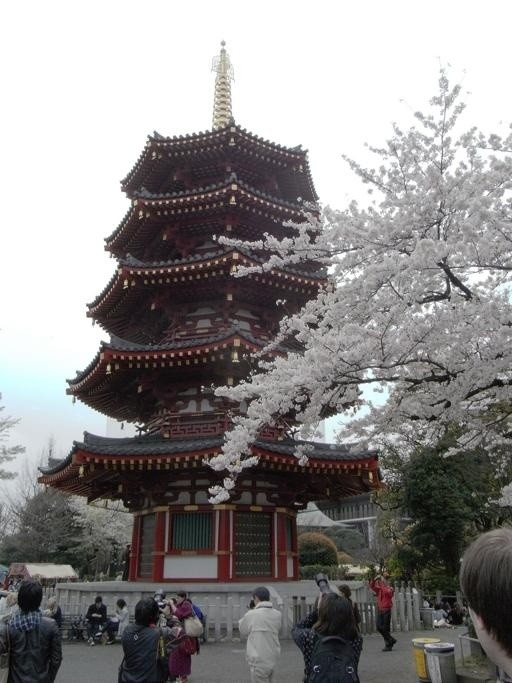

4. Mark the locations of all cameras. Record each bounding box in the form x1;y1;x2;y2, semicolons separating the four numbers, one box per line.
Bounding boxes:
315;573;330;592
374;575;381;580
249;600;255;609
153;589;167;609
166;597;176;606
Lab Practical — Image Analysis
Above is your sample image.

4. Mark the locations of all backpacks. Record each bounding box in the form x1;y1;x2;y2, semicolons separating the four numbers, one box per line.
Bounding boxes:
308;632;359;683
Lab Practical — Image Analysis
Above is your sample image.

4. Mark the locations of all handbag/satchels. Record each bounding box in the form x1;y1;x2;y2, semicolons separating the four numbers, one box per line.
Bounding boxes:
184;615;204;637
157;655;168;683
0;652;10;683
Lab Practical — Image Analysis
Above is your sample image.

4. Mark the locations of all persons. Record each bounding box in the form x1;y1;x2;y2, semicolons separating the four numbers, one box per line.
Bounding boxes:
291;575;397;682
1;576;63;682
238;587;282;681
458;526;512;682
422;598;469;629
86;591;206;682
120;544;131;580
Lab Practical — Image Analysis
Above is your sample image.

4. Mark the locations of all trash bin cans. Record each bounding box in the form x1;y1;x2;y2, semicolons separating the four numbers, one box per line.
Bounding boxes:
420;608;435;630
425;642;457;683
413;637;441;679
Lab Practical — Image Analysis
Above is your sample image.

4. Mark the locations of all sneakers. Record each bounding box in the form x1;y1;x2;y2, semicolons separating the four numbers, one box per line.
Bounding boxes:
383;638;396;651
